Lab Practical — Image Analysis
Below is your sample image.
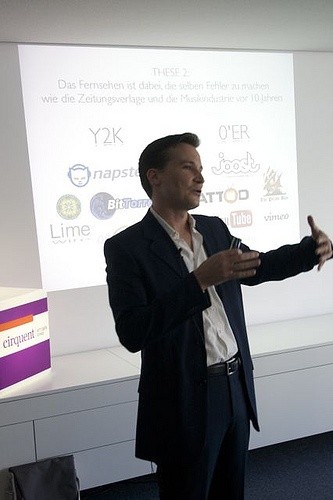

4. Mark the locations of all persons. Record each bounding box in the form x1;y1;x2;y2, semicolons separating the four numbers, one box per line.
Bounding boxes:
104;132;333;500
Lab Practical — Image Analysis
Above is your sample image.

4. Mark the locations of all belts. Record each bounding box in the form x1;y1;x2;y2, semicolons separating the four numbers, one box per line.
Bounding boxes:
207;357;242;377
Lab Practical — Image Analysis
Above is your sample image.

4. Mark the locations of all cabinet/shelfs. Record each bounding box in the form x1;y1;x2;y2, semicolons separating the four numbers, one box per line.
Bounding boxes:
0;312;333;491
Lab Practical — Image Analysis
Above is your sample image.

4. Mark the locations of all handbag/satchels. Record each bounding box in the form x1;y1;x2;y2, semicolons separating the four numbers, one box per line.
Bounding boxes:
8;455;81;500
